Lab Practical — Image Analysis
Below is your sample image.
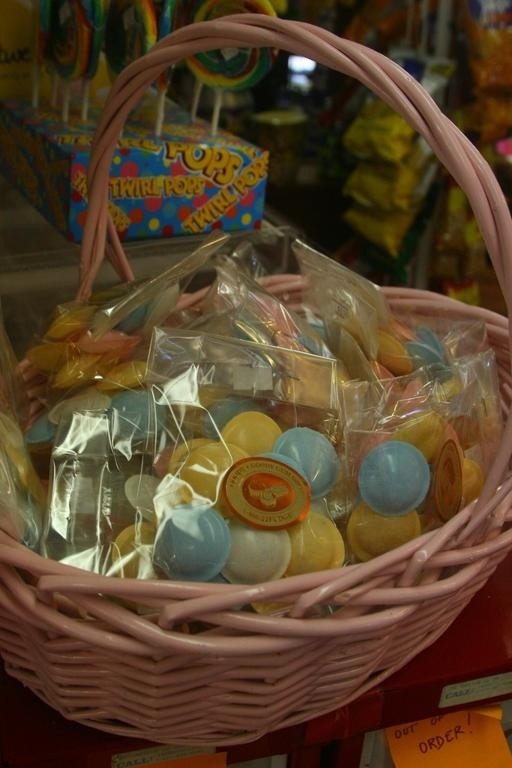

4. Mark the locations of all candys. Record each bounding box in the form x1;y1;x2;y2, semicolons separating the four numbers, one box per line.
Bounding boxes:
31;0;280;137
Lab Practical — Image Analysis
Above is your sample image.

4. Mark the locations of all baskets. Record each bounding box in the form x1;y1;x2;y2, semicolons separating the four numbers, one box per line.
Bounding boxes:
1;13;512;748
